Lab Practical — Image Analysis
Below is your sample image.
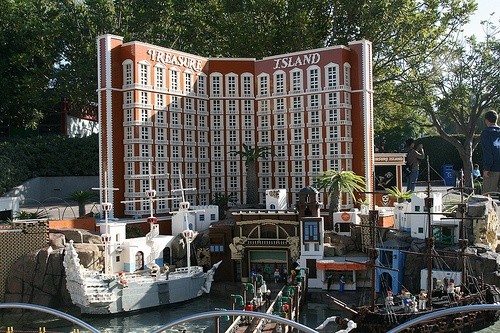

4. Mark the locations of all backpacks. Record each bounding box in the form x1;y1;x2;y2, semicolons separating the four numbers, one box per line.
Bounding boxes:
404;164;412;176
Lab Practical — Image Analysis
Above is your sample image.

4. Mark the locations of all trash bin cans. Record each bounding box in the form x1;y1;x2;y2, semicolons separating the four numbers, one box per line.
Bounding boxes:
443;165;456;187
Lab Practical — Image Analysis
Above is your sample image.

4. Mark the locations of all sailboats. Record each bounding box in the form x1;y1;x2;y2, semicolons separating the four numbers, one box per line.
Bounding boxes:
326;155;500;333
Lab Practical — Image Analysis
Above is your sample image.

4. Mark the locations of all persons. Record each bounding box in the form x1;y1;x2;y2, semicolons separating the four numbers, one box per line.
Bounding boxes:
326;274;333;289
397;289;406;309
403;292;414;313
385;291;395;314
339;276;345;292
480;110;500;196
411;296;418;313
243;301;252;320
273;267;280;283
406;138;425;192
283;302;289;313
151;265;158;280
163;263;170;280
473;164;481;182
118;271;128;289
418;289;428;309
446;278;457;304
265;290;271;310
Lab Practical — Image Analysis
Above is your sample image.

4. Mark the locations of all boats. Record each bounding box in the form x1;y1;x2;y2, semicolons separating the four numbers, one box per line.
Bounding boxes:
62;157;223;316
